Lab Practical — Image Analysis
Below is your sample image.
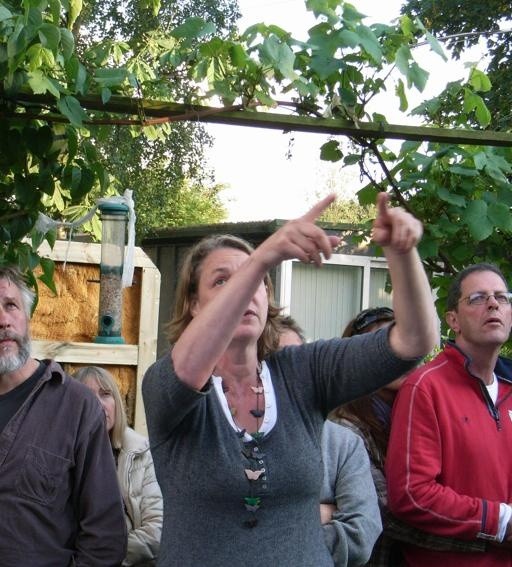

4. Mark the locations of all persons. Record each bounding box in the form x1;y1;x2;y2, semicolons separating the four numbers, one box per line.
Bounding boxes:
271;313;384;567
1;260;128;567
69;365;164;567
141;192;439;567
385;263;512;566
327;303;486;567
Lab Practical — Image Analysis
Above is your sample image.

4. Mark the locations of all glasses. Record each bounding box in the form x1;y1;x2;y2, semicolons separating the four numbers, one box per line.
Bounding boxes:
453;290;512;306
352;308;395;331
